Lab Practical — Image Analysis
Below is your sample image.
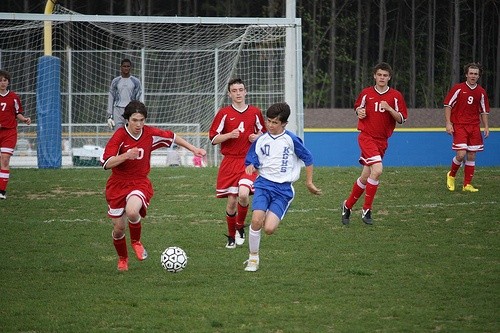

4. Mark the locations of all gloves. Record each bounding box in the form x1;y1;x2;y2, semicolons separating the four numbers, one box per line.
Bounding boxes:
108;118;116;130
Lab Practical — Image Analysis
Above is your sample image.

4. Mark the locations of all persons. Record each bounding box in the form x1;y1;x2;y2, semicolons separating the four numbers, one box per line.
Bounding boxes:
209;79;268;249
444;63;489;192
106;58;142;132
100;100;207;271
342;63;408;227
0;70;30;200
244;102;322;272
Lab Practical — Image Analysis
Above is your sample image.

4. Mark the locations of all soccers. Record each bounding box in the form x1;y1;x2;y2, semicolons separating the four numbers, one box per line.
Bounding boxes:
161;246;187;273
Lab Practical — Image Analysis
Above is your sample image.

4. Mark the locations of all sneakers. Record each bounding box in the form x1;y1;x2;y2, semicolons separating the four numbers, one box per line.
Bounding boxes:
446;171;457;192
0;190;7;200
226;228;246;248
462;183;479;193
241;254;260;272
131;240;149;260
341;200;351;226
360;207;374;225
118;257;128;272
